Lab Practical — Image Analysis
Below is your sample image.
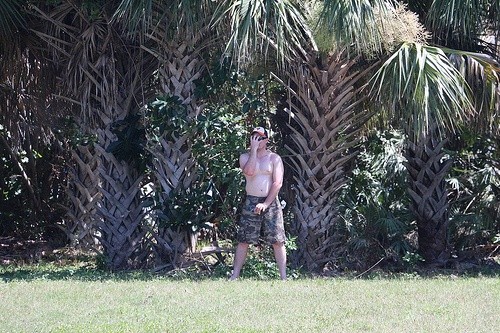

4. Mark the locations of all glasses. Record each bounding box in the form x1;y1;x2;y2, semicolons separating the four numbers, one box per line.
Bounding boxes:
252;137;267;141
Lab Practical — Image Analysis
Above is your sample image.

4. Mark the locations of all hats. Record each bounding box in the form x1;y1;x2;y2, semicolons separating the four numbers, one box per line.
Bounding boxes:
251;127;268;138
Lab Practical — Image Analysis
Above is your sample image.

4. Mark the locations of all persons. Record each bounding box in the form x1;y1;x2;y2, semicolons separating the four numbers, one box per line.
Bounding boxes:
231;127;286;280
